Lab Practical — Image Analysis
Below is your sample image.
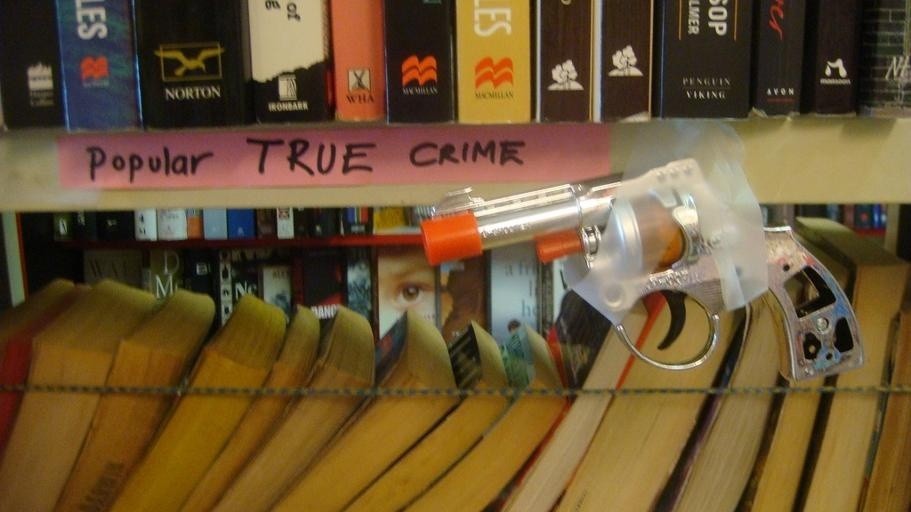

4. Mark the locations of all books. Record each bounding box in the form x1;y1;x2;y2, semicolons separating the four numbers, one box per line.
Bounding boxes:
0;0;909;138
1;203;908;512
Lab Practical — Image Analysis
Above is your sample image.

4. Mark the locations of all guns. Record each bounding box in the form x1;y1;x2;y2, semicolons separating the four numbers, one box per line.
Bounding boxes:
414;159;866;381
